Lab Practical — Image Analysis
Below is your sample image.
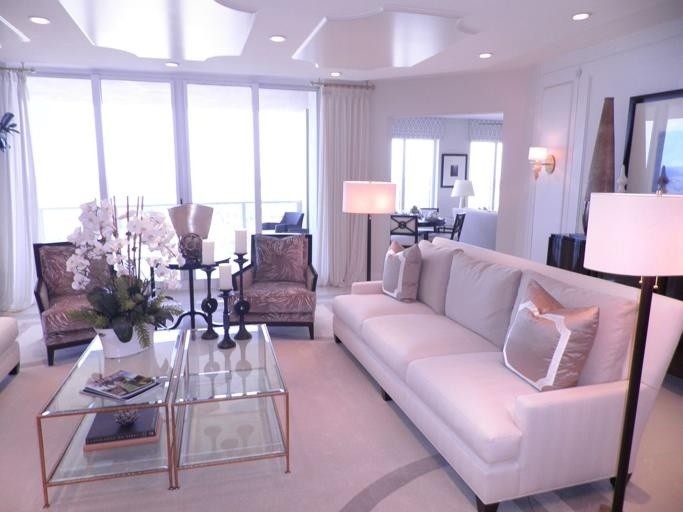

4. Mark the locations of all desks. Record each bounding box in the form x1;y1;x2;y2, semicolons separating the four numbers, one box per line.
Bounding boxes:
416;215;446;232
151;255;231;329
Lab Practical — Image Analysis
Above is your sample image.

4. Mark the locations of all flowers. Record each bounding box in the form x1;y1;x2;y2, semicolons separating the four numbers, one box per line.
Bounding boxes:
66;195;184;348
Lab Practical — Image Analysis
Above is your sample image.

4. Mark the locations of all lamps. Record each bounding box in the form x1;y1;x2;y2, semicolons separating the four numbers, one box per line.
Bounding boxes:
291;9;472;72
583;192;683;512
450;180;475;207
342;181;396;281
59;0;256;63
529;146;556;178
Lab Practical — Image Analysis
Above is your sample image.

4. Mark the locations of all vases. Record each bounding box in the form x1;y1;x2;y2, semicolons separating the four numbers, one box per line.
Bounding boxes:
94;318;154;358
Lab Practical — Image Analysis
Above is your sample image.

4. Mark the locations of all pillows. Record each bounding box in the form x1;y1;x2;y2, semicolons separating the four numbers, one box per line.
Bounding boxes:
506;268;637;385
382;239;422;303
500;280;599;391
445;251;521;349
416;239;464;315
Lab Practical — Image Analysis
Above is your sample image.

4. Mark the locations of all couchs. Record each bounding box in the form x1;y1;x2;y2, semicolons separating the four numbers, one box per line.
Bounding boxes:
0;317;19;384
262;211;304;233
227;234;318;340
331;235;683;511
33;242;93;366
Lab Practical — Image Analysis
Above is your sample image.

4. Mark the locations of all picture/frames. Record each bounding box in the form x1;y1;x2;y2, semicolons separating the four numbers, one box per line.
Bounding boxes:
441;154;468;187
620;88;683;192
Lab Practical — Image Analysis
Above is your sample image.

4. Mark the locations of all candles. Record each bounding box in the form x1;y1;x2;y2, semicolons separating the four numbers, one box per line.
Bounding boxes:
235;231;247;253
202;239;215;265
219;263;232;289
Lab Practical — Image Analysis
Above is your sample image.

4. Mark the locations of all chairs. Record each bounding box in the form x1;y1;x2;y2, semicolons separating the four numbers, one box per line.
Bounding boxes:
420;208;438;217
390;215;418;248
426;212;465;241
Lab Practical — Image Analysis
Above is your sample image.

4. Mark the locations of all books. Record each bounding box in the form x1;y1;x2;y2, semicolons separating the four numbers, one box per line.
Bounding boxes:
83;412;162;452
81;367;158;401
84;406;159;444
